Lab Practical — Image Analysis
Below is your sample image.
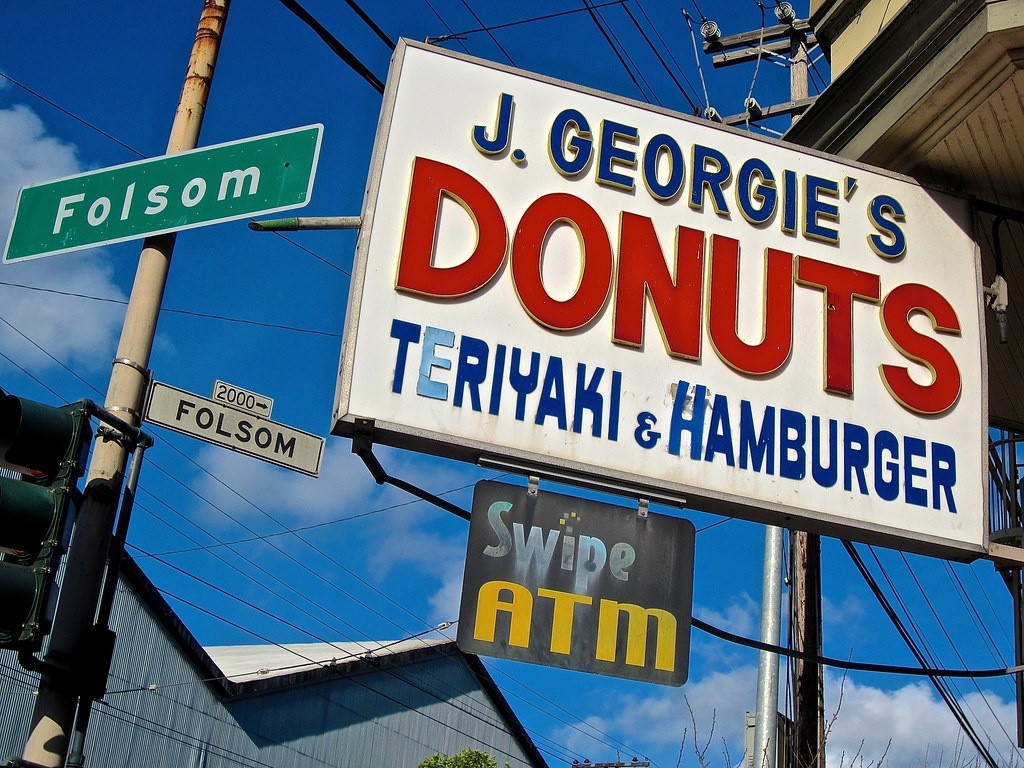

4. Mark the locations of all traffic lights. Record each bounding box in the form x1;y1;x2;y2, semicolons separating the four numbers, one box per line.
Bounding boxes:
0;393;96;655
